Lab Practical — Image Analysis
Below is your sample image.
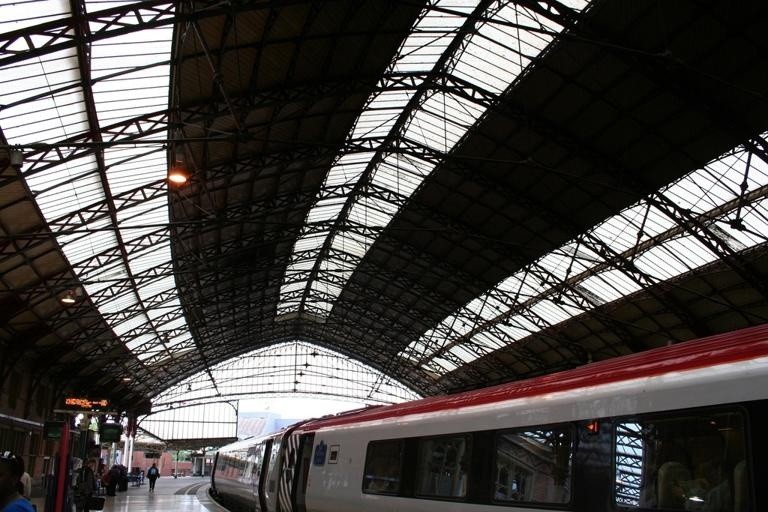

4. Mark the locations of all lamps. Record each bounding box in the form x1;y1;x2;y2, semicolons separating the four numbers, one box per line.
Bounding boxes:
123;374;132;381
60;288;76;303
168;153;186;183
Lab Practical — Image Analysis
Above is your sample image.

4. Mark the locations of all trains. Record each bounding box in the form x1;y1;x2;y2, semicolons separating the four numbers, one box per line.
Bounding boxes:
210;324;767;512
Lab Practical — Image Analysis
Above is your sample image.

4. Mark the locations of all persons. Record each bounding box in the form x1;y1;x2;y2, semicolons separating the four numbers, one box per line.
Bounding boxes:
147;463;160;492
0;450;128;512
